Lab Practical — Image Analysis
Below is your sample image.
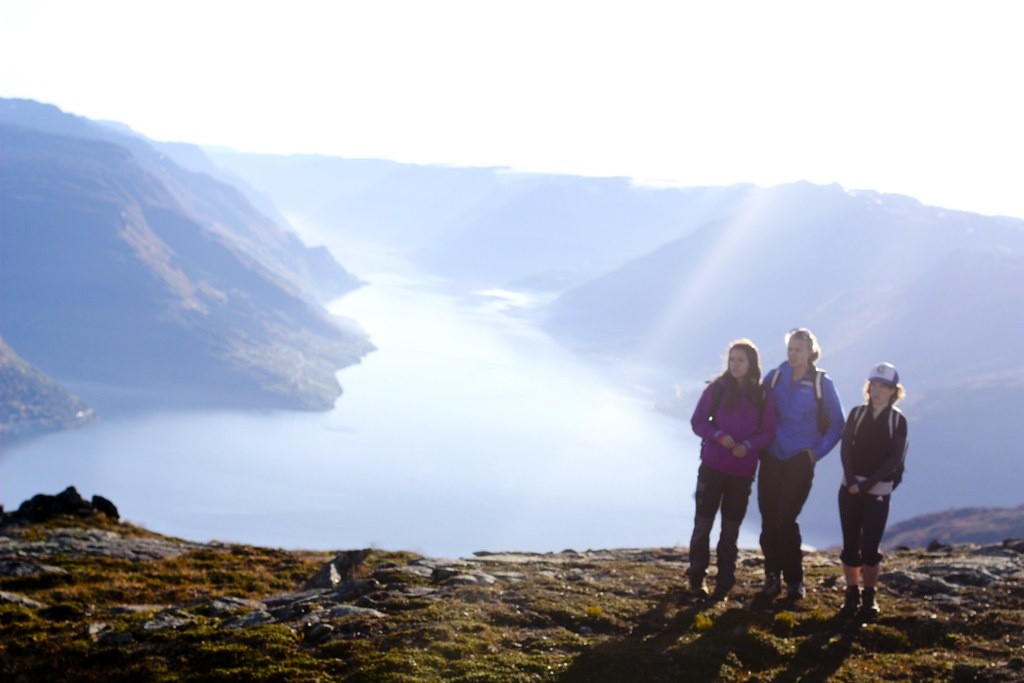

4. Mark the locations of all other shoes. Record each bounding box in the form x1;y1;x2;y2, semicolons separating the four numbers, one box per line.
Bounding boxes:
862;590;881;616
842;588;861;618
754;571;782;599
711;587;728;602
685;575;709;595
783;575;806;599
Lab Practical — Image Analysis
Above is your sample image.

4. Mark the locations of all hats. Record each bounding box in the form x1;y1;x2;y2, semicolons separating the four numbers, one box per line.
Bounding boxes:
868;361;900;386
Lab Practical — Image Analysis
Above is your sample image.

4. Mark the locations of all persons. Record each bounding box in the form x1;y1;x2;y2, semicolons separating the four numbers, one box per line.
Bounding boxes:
755;330;845;599
685;342;778;601
838;362;909;621
